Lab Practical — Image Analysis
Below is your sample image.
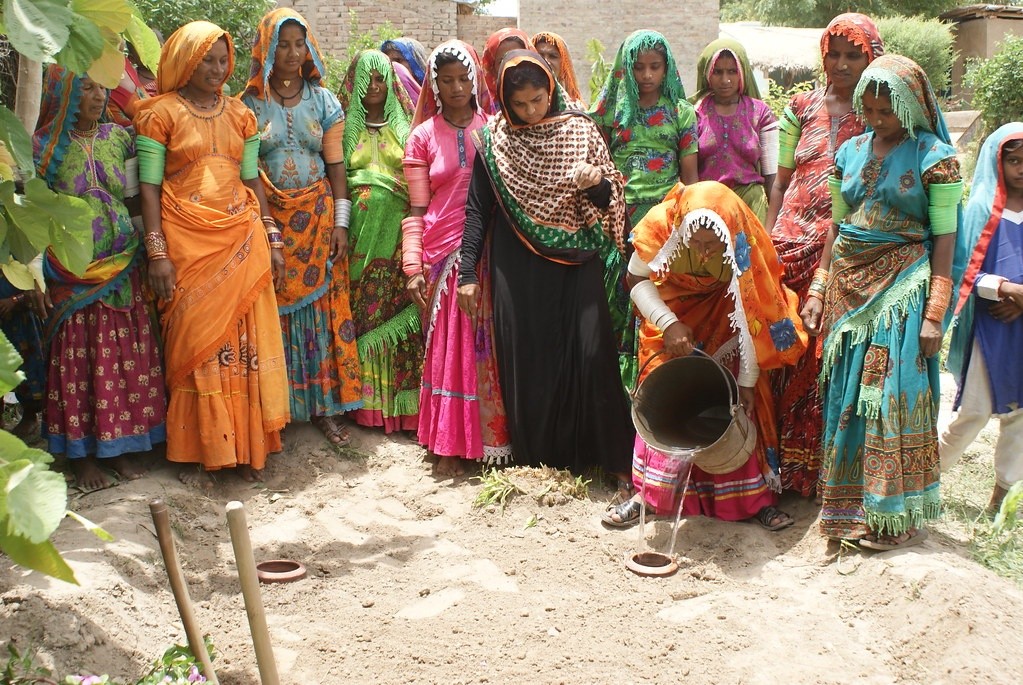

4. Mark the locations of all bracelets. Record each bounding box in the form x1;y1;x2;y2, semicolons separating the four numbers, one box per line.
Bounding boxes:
807;268;828;301
922;275;955;323
262;215;285;249
13;292;26;304
144;230;170;260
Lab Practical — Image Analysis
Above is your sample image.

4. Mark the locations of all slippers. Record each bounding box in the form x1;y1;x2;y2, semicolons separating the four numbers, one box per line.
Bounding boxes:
755;506;794;531
314;413;354;448
858;521;928;551
613;471;635;502
602;497;670;526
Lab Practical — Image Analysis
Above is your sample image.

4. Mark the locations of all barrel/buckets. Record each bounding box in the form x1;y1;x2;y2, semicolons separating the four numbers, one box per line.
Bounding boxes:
625;348;757;475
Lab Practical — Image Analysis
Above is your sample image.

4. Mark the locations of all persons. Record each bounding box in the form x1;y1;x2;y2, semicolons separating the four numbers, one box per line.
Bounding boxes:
583;29;698;404
239;8;366;446
762;13;886;507
102;26;165;130
132;20;292;488
336;48;423;439
480;27;567;119
0;269;48;439
456;49;625;484
30;60;166;490
797;55;965;547
937;120;1023;519
600;181;809;530
400;36;515;476
380;37;428;85
530;32;582;100
685;38;781;233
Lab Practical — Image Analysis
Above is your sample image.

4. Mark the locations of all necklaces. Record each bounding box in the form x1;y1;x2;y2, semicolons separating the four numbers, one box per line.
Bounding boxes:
273;73;300;87
686;245;725;286
443;117;466;129
362;118;388;137
714;97;739;107
271;80;304;108
178;89;218;110
